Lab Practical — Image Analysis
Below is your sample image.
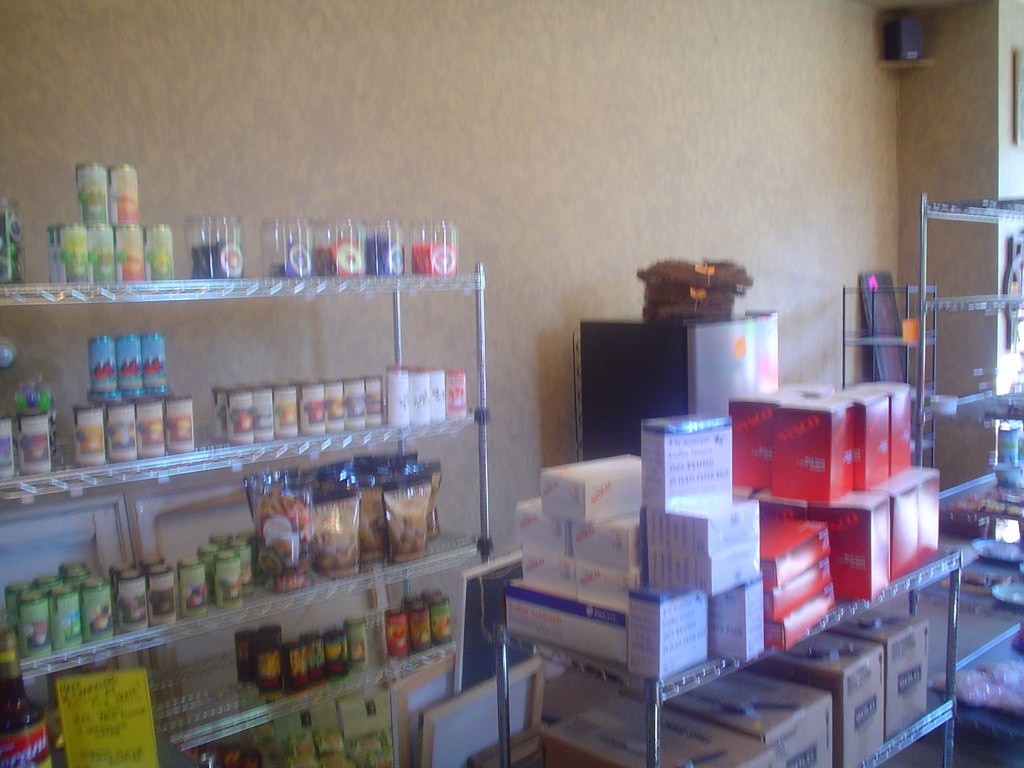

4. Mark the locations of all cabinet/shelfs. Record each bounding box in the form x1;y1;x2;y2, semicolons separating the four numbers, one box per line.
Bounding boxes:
916;190;1024;736
0;260;493;767
842;283;936;468
496;545;966;767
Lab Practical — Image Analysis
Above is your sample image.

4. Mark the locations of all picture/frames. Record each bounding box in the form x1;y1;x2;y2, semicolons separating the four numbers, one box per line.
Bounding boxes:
391;552;545;768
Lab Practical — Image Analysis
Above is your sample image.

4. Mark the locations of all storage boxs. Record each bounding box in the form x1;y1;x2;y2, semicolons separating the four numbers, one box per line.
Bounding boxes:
502;380;949;679
540;696;774;767
828;610;928;740
752;630;885;766
664;668;832;767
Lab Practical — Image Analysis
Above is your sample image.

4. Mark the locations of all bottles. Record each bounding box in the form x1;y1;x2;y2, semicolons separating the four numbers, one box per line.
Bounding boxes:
0;627;53;768
365;218;405;277
315;217;370;281
185;213;246;281
411;216;462;278
260;217;315;281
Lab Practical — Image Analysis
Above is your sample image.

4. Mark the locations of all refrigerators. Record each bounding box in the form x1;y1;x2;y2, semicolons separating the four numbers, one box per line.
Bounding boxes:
579;309;781;463
